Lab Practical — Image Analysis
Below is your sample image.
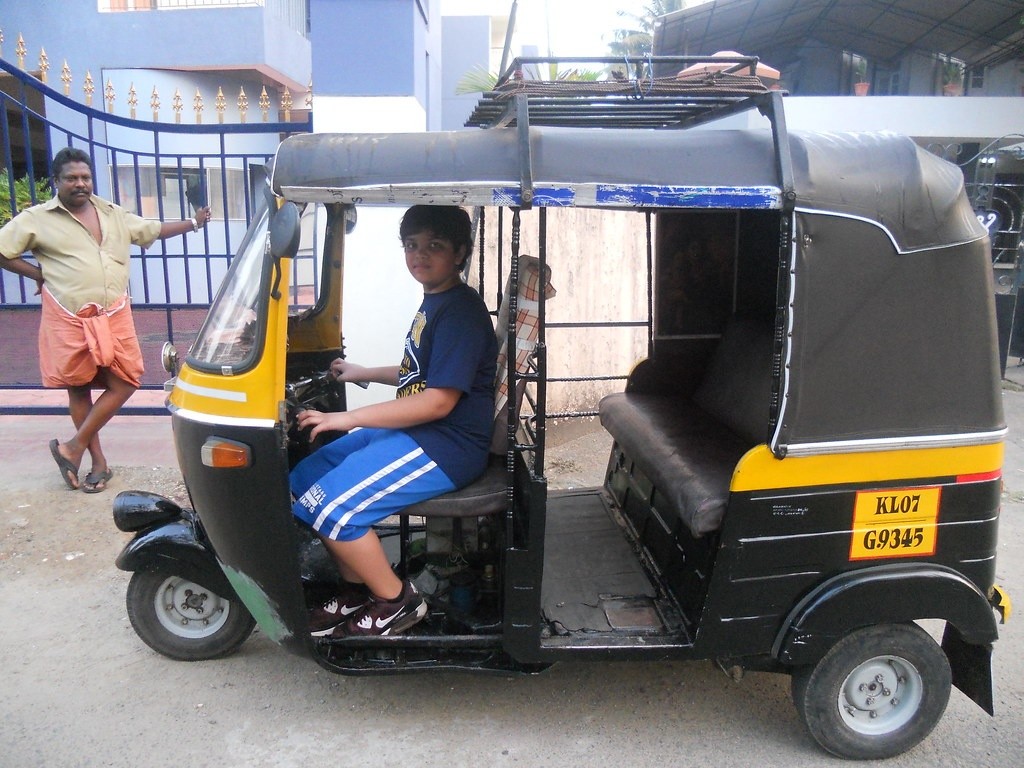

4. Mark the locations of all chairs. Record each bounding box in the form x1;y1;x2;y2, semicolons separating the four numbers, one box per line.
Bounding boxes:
390;254;552;609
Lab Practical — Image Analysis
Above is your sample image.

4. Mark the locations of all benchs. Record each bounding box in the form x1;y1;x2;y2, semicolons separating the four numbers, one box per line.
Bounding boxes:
598;314;776;643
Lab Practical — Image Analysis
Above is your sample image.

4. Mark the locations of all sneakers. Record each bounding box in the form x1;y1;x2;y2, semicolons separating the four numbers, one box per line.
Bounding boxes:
306;581;372;636
331;581;427;637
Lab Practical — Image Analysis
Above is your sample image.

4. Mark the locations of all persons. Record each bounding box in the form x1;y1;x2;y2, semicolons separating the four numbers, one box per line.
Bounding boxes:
0;146;211;494
289;204;500;637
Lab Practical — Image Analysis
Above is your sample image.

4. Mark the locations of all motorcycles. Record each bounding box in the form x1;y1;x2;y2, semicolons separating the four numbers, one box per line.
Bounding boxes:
112;51;1012;763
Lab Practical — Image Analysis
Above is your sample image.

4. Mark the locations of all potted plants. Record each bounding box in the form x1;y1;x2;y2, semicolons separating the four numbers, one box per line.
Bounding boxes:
855;57;871;96
943;63;963;96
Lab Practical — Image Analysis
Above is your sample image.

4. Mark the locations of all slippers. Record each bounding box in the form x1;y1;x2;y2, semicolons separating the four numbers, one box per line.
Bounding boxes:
83;468;113;493
49;439;81;490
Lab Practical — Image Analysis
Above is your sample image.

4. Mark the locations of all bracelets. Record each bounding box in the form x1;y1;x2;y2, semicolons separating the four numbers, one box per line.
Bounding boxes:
190;217;198;233
